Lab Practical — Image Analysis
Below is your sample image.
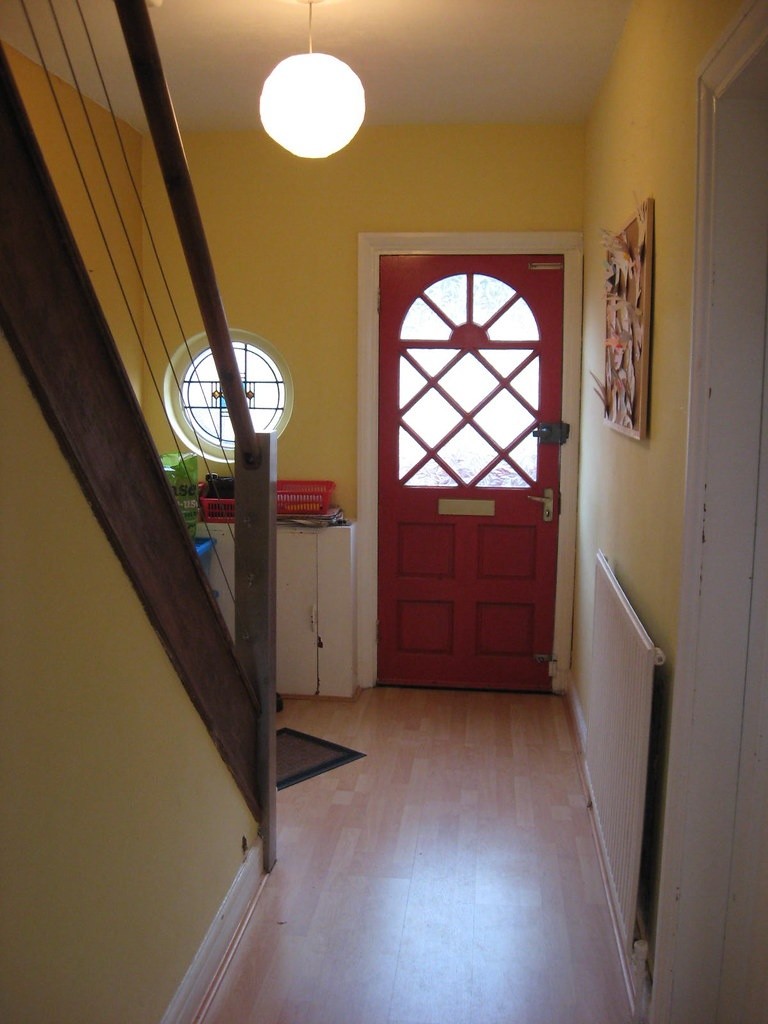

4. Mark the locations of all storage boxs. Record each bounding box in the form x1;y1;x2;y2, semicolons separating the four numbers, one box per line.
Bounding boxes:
200;492;235;522
277;480;336;514
195;536;217;574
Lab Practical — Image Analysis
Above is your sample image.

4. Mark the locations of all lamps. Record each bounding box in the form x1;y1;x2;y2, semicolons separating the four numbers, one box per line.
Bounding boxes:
259;0;367;159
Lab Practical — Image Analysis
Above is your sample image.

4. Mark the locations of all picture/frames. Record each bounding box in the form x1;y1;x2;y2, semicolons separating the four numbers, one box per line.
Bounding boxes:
586;197;653;441
